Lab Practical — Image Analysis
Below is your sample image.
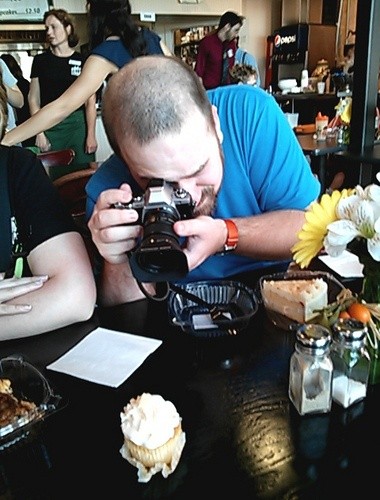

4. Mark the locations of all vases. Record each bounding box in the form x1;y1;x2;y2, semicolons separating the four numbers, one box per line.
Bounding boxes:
343;267;380;386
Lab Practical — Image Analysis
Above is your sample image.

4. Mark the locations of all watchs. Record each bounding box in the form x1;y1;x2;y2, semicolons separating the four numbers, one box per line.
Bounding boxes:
215;219;239;256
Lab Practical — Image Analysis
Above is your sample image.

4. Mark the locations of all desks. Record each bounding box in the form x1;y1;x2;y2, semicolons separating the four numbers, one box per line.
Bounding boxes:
272;93;336;114
0;252;380;500
296;117;380;204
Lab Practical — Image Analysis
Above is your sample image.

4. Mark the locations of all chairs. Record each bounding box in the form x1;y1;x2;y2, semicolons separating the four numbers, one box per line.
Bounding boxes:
36;147;106;269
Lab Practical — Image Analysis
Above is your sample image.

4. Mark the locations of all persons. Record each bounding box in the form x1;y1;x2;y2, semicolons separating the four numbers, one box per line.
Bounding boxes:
193;11;260;92
86;53;320;307
0;71;96;341
0;0;172;182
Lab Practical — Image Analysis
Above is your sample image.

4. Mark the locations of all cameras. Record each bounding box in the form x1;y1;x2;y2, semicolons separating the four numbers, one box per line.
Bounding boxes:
111;177;197;283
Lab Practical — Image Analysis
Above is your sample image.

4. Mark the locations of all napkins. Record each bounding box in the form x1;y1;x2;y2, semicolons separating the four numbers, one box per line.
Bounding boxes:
46;325;162;389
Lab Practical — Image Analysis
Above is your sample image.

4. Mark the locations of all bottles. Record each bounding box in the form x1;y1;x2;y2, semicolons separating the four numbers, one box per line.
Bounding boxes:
330;316;371;409
338;124;349;145
289;324;335;418
300;68;308;93
315;112;328;141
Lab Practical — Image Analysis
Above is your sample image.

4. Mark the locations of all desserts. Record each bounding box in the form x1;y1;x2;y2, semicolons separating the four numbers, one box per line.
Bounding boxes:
120;393;182;469
260;278;327;324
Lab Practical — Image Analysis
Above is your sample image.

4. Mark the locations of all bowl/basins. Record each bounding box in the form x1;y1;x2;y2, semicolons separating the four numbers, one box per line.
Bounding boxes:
0;354;62;448
258;268;345;331
167;278;261;338
278;78;297;93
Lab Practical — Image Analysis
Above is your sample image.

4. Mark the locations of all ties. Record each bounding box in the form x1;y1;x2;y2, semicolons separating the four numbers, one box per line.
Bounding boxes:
220;46;229;87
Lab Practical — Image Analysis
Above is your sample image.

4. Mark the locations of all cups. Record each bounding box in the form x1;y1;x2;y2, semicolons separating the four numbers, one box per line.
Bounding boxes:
316;82;325;94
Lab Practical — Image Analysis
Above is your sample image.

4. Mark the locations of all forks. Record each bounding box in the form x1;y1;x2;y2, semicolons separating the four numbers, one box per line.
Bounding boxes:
168;282;230;324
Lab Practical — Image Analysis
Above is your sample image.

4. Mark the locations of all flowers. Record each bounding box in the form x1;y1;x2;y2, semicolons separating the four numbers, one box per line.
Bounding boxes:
289;171;380;270
